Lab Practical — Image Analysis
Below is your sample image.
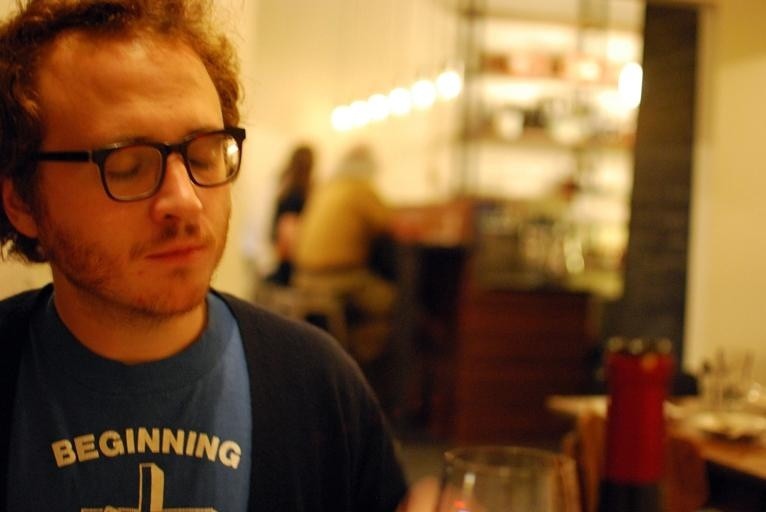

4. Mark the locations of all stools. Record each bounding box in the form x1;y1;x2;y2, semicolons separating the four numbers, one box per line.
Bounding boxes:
271;290;350;352
254;282;288;311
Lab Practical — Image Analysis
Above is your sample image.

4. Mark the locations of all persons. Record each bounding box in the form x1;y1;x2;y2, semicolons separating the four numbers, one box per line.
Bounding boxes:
293;137;403;370
261;142;315;292
0;1;418;512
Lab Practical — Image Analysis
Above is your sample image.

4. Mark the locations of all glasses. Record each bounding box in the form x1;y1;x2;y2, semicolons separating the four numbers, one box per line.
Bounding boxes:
37;126;248;204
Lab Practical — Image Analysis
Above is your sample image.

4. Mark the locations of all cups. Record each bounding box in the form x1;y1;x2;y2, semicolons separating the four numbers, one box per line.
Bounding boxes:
435;445;582;511
601;339;674;481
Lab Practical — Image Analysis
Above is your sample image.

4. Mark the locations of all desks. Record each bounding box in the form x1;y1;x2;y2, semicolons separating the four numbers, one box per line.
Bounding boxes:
545;393;766;512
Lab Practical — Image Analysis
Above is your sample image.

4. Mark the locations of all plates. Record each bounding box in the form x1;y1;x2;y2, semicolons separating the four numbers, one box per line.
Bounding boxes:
692;411;765;439
